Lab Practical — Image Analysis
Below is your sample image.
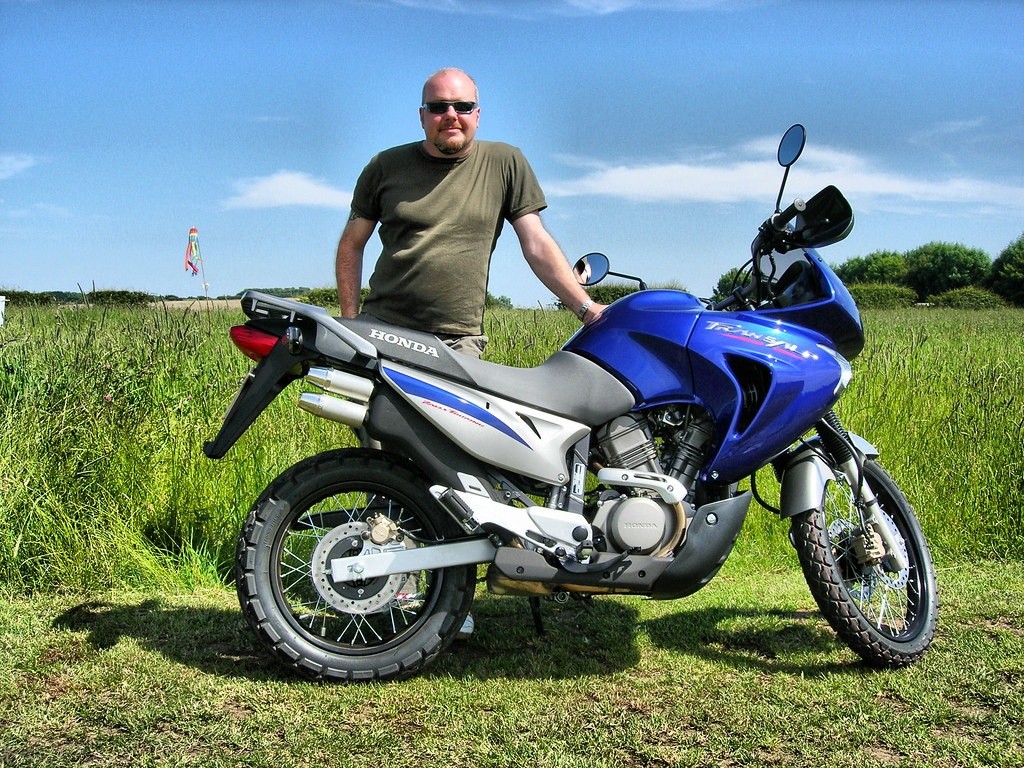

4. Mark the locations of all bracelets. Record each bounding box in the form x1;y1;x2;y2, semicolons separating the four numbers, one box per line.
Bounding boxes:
577;298;595;321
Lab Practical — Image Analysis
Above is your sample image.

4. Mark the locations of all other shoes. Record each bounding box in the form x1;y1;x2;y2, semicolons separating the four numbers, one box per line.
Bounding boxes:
391;589;426;611
457;612;474;639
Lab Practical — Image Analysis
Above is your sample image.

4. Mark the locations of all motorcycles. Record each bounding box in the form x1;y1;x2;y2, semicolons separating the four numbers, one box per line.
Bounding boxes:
201;122;941;687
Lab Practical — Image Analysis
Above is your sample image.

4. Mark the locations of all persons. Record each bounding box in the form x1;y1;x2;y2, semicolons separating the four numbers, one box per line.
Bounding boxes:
335;67;613;635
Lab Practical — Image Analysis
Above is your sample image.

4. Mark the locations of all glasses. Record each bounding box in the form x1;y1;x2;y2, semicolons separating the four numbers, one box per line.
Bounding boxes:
421;101;478;115
